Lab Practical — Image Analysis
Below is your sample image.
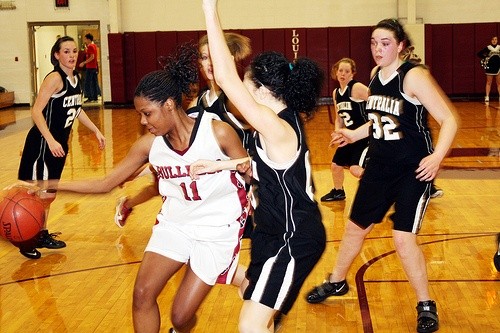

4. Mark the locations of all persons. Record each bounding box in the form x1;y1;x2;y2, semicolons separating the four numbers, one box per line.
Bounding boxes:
320;58;372;202
2;39;252;333
306;19;459;332
119;32;254;287
203;0;326;333
17;36;106;259
477;36;500;102
79;33;101;104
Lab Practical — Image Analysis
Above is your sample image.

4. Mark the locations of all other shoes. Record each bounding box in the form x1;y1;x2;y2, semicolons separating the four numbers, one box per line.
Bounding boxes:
84;99;98;103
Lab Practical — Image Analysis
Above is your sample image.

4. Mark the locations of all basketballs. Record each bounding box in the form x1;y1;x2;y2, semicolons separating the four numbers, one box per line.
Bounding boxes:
0;188;46;242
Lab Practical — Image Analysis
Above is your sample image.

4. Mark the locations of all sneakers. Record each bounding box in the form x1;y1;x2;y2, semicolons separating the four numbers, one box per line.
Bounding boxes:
34;232;66;249
20;246;41;259
113;196;133;229
423;184;444;199
307;274;349;304
320;185;346;202
416;300;439;333
